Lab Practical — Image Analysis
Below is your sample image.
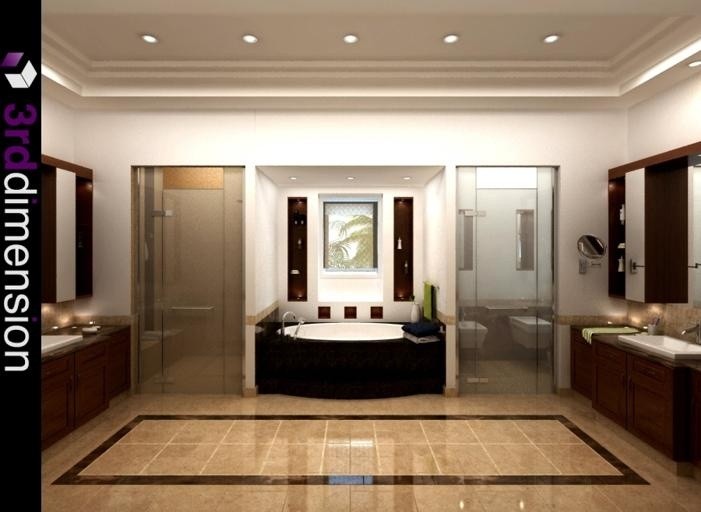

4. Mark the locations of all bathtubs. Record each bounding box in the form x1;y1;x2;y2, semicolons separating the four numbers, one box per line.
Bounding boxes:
276;319;409;341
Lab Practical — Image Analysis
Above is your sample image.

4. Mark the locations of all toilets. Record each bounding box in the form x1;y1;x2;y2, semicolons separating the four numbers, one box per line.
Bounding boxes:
510;315;552;366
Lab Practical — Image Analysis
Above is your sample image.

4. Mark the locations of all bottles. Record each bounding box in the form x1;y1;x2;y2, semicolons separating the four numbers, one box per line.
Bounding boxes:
410;303;421;324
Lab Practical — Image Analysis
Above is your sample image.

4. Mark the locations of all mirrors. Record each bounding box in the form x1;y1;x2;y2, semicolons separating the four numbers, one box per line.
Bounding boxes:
578;234;607;268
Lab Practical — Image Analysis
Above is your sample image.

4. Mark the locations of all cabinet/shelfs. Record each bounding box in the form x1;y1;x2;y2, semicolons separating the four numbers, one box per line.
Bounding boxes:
41;340;110;451
570;328;593;399
109;325;131;398
607;159;690;306
40;155;95;303
591;335;690;461
687;368;700;467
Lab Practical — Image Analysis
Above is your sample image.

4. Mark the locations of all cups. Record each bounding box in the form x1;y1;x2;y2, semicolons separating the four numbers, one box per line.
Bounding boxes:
647;324;658;336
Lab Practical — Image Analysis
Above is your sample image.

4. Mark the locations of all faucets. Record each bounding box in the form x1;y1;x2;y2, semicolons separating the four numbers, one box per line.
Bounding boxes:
280;311;296;335
461;308;466;323
681;320;701;344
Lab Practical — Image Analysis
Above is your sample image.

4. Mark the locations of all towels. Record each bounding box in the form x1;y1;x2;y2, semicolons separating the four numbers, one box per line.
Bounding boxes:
402;321;440;345
583;326;641;344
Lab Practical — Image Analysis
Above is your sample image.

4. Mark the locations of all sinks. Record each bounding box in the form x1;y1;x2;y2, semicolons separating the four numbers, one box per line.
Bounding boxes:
653;345;701;361
459;321;489;349
40;333;82;354
617;334;701;345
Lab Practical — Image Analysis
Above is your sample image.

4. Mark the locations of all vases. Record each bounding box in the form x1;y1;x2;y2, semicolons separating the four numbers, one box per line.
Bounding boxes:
411;303;421;322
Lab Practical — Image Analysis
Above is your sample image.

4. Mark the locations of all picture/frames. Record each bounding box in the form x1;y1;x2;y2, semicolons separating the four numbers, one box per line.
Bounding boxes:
317;190;384;279
